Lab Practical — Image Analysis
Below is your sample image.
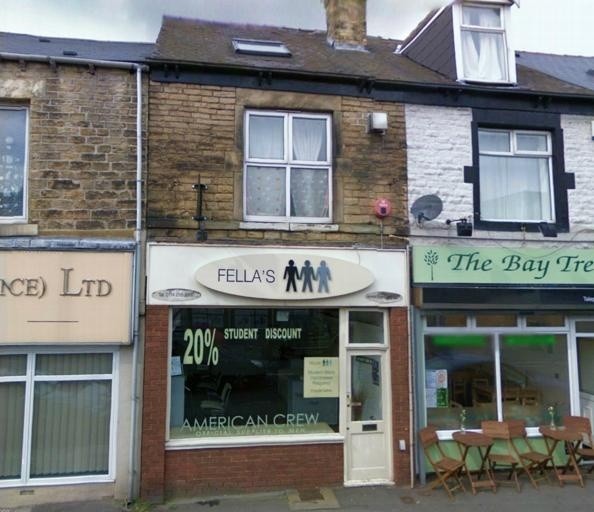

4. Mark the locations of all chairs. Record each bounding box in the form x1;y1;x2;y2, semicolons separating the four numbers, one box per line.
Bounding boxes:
439;370;541;414
415;415;594;501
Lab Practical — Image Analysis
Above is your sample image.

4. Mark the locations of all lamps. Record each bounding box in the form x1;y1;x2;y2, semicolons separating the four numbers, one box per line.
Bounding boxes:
521;222;557;239
445;218;473;237
